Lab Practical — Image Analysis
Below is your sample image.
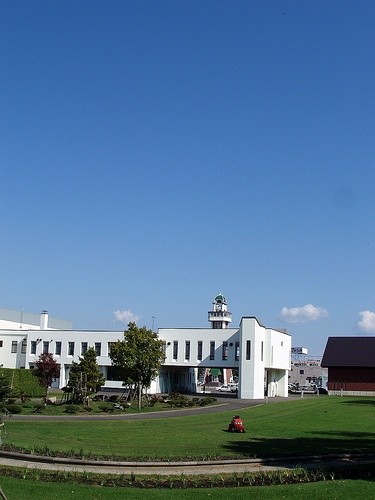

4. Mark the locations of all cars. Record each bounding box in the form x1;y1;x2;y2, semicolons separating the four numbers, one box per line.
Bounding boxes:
214;381;317;393
